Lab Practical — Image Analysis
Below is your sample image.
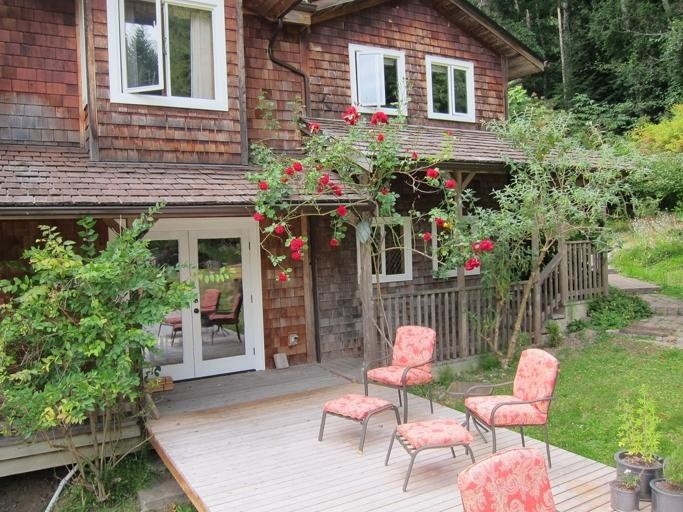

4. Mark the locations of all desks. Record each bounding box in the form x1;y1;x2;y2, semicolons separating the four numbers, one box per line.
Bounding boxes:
446;381;493;444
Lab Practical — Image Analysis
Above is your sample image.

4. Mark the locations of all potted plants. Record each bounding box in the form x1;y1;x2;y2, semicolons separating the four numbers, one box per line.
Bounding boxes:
606;383;683;512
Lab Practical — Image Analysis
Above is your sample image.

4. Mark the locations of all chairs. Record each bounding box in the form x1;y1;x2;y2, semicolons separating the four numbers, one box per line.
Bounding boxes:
456;446;557;512
463;349;560;468
156;287;241;346
362;323;436;421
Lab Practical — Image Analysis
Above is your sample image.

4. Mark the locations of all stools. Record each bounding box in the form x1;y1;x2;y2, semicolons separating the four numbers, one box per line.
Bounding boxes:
320;394;475;492
